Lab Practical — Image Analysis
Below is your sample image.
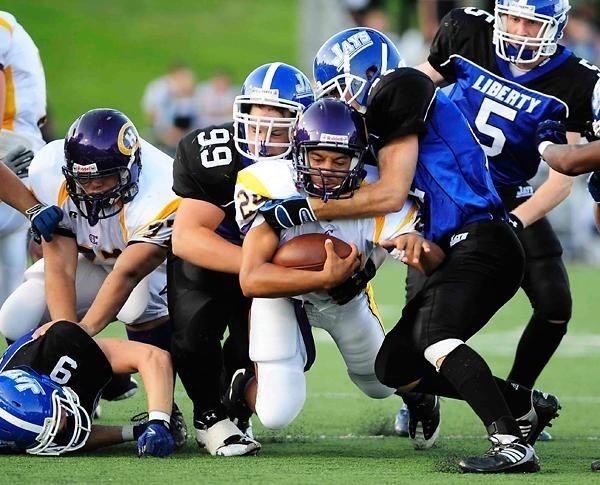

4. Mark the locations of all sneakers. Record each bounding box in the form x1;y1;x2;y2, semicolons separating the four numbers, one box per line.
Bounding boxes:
192;406;262;457
218;362;256;442
456;437;542;474
511;387;563;449
407;390;443;451
168;403;187;448
393;401;410;436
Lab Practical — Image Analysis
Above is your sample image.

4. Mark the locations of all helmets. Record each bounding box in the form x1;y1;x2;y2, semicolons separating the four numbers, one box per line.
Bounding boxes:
290;97;369;201
0;367;92;456
493;0;572;66
58;106;142;225
311;24;408;119
231;62;314;163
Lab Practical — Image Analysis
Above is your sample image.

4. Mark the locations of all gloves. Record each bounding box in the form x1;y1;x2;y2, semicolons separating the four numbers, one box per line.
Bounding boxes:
535;120;566;160
25;204;64;243
134;410;177;459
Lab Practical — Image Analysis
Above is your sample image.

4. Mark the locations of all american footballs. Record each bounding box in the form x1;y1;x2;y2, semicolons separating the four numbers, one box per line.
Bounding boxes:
271;233;352;271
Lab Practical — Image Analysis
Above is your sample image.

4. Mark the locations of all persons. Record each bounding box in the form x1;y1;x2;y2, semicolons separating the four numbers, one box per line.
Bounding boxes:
409;0;599;451
268;26;562;473
534;118;599;473
0;9;48;304
166;62;315;459
0;108;186;450
141;63;241;156
0;318;177;458
292;0;600;269
1;144;64;243
219;97;446;437
